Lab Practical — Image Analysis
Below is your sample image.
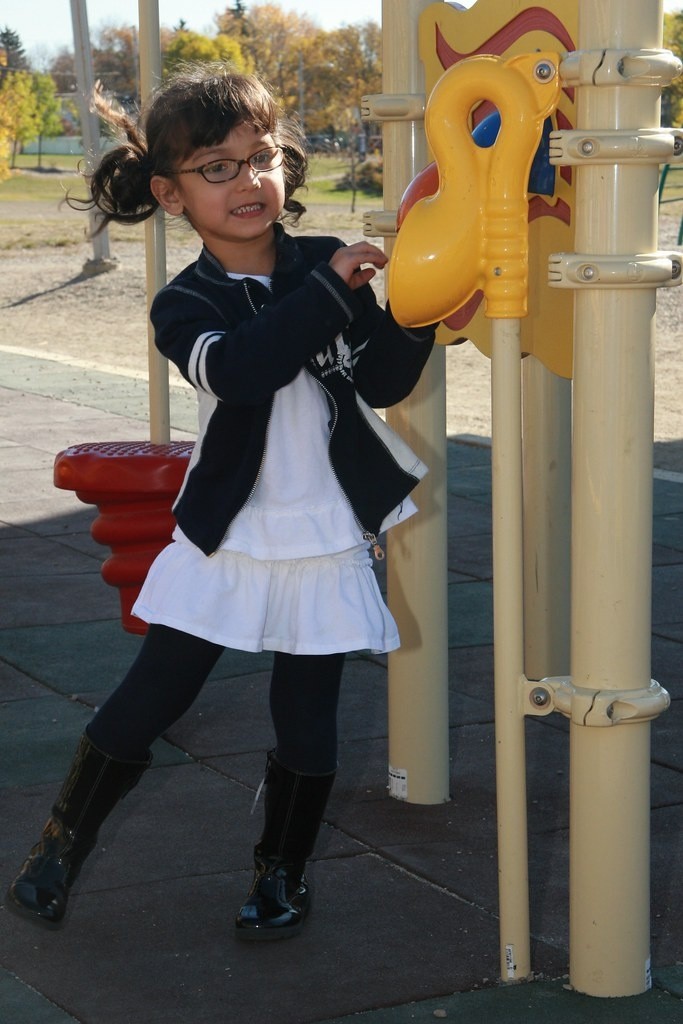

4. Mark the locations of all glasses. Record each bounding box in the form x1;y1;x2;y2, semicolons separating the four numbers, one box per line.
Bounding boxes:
163;145;285;183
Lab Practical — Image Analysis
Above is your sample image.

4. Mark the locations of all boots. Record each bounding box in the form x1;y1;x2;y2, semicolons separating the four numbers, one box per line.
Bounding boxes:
235;750;340;939
6;721;154;931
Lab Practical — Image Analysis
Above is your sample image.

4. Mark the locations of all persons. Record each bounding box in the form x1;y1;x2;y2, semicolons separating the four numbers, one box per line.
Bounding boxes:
5;64;438;943
